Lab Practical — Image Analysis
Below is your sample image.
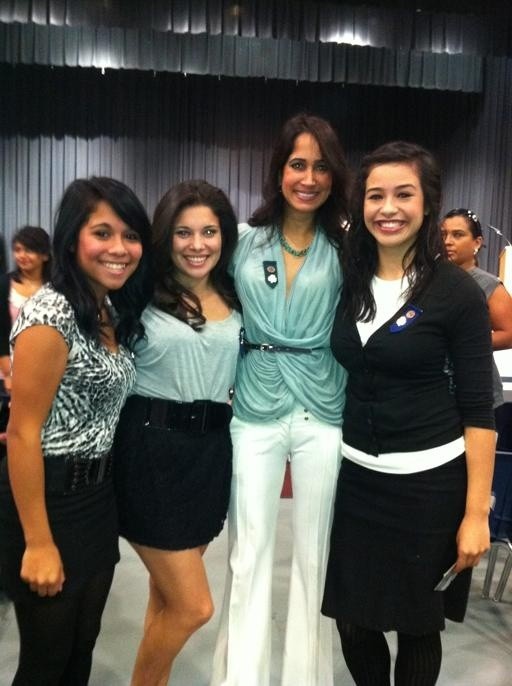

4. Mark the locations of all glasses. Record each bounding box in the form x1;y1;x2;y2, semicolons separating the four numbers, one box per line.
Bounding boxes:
445;206;480;229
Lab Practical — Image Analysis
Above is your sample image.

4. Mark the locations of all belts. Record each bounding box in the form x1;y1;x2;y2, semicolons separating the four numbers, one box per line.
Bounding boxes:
44;453;114;496
241;342;329;354
123;391;227;433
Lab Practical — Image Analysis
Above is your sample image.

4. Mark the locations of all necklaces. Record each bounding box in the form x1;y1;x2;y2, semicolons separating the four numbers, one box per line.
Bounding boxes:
275;226;317;256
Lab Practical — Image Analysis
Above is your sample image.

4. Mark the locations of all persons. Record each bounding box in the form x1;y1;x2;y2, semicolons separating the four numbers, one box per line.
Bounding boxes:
115;182;246;686
0;226;57;433
209;112;354;686
435;206;512;456
0;176;156;686
0;231;15;396
325;142;501;686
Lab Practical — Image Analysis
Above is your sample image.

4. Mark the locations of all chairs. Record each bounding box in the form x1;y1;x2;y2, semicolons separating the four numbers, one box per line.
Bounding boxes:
482;402;511;600
